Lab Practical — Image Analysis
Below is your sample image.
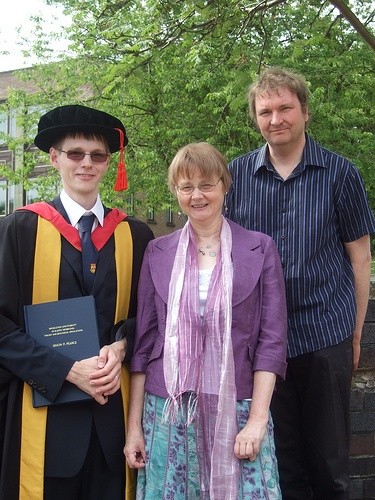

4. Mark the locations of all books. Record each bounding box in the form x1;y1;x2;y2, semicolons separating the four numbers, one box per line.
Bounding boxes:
23;295;101;408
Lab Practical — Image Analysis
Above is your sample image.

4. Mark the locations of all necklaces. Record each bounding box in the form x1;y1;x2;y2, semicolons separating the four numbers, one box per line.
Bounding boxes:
197;245;220;256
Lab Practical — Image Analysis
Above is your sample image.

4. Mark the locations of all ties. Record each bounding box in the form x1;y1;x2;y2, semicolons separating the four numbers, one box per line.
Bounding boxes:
79;214;97;294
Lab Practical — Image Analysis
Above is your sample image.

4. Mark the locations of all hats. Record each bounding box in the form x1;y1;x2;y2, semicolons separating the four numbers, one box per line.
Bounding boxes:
34;105;129;192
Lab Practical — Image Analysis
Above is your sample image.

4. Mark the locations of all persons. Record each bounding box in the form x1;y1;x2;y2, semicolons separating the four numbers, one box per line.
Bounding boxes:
221;68;375;500
0;105;156;500
123;141;287;500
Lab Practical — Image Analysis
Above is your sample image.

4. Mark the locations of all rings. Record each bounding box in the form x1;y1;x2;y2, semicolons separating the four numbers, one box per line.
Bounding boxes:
114;375;119;380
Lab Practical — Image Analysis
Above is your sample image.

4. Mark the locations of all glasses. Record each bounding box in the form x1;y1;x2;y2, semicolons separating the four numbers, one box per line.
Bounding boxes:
52;147;111;163
175;178;221;196
